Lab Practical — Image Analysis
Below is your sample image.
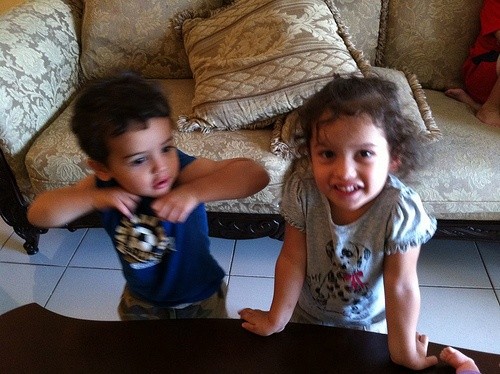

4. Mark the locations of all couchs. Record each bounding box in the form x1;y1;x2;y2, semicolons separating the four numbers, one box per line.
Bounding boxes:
0;0;500;260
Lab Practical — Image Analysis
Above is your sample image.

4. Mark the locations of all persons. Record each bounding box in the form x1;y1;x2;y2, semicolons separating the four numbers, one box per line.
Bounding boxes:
27;73;270;321
440;346;481;374
236;75;438;369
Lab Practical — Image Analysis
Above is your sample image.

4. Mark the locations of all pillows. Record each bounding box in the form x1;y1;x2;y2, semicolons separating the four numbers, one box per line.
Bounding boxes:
169;0;382;140
269;66;441;160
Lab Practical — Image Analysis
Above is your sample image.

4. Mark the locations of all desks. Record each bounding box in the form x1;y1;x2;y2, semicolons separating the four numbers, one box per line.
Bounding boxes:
0;301;500;374
